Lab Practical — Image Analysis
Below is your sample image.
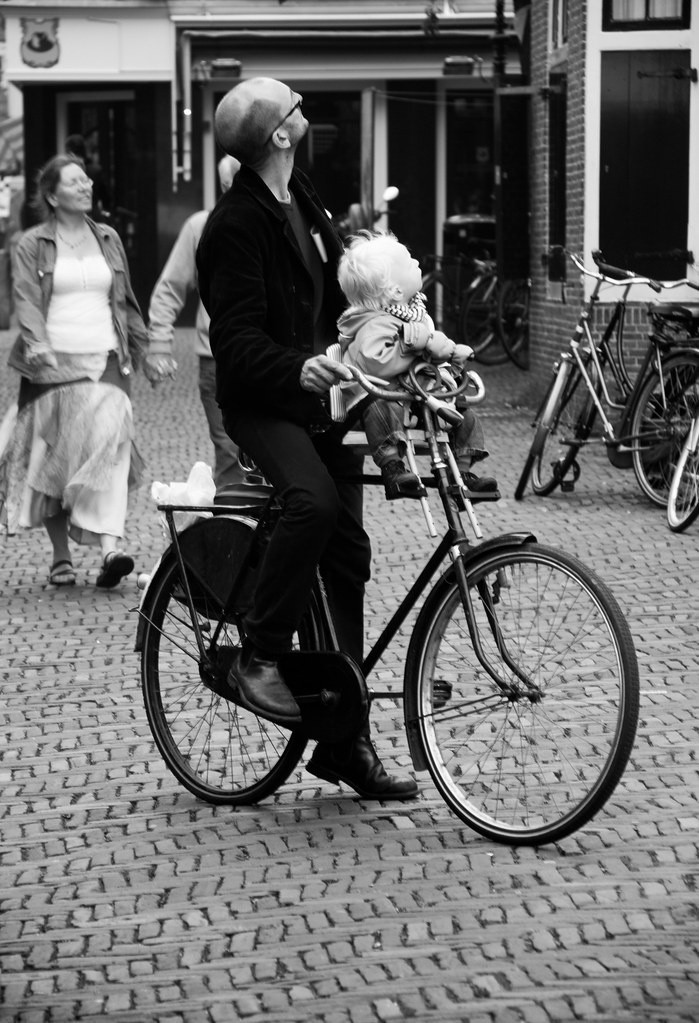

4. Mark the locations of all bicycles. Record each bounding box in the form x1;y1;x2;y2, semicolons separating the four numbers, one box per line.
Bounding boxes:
514;245;699;533
132;363;639;844
417;240;529;371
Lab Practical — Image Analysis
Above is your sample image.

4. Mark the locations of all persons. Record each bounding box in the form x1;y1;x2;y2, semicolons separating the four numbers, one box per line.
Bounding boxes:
0;152;149;588
338;233;497;511
150;152;246;517
197;77;420;800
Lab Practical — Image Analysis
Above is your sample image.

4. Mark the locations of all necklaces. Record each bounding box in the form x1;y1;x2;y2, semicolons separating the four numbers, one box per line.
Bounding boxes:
55;230;88;249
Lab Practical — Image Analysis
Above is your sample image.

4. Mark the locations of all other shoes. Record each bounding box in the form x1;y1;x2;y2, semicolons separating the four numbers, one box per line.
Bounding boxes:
384;461;420;501
455;471;497;510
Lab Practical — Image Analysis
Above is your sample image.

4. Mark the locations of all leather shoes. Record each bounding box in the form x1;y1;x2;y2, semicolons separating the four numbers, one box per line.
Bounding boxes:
306;738;421;802
225;649;300;724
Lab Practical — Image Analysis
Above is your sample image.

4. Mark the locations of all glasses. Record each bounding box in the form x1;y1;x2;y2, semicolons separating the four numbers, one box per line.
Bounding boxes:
263;100;304;148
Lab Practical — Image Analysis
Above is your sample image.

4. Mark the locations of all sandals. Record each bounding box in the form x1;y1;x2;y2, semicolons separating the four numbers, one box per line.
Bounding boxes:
49;560;76;586
96;551;134;590
